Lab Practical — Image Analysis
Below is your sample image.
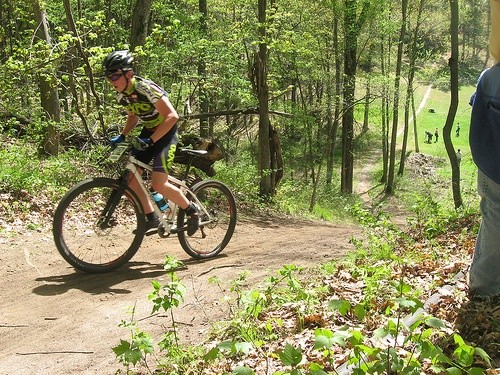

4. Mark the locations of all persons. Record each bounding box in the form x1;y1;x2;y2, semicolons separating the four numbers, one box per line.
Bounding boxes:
103;51;200;236
425;131;433;144
467;62;500;299
456;149;462;164
456;123;460;137
435;128;438;142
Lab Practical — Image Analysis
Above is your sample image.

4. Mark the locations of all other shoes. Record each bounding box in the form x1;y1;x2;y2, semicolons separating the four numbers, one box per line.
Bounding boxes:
187;202;201;236
132;218;163;234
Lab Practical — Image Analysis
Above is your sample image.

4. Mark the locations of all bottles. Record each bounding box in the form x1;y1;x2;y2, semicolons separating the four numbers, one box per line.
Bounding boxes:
149;188;169;211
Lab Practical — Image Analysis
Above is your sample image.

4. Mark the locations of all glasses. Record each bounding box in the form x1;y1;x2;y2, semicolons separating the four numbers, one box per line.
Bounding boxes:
106;71;128;81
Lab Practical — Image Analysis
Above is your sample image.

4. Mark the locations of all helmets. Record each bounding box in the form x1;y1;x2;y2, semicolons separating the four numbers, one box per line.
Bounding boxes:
102;50;134;76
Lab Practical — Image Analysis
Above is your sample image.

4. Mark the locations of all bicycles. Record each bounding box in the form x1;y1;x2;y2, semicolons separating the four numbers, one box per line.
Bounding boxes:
51;139;238;274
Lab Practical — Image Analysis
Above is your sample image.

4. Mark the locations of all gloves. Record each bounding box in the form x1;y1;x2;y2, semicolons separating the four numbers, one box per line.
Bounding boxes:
110;133;125;150
134;137;154;151
